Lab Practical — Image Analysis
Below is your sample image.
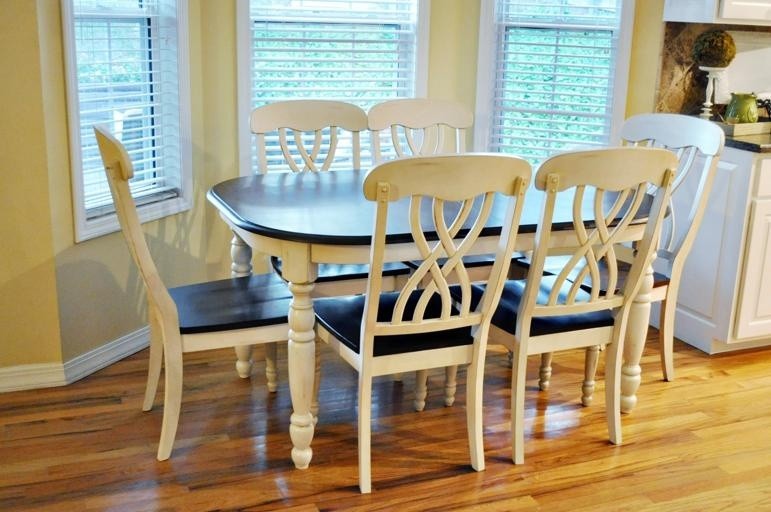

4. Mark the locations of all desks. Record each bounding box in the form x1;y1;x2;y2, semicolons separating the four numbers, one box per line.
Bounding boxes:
207;169;670;472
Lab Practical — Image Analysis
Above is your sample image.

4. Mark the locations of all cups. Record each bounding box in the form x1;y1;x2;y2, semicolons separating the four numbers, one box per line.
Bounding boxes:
728;112;740;125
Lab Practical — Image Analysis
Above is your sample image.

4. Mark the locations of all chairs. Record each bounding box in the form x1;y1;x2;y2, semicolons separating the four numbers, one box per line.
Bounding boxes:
92;124;320;462
445;148;676;465
313;153;532;493
249;99;409;382
368;98;525;289
518;113;724;405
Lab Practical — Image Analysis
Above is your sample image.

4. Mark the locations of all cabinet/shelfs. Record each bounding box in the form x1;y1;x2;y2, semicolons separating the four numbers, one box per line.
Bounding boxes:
650;145;771;355
663;0;770;27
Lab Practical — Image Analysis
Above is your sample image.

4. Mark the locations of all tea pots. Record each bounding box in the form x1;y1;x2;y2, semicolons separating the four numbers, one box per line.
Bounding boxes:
725;91;758;123
